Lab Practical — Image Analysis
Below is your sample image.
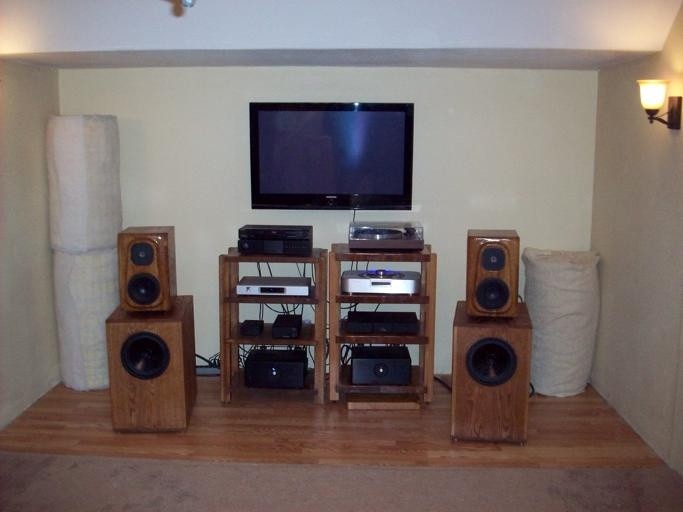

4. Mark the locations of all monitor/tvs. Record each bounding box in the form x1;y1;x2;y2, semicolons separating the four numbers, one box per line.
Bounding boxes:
249;102;414;209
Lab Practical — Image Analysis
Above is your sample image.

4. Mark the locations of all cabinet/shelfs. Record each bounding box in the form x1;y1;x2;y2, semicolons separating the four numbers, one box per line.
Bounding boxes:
219;247;328;405
328;243;437;402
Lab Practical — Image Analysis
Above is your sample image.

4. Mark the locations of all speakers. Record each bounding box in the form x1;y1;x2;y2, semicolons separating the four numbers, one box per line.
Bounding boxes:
243;348;305;389
117;225;179;314
450;300;534;445
465;229;520;319
105;295;196;433
351;346;412;387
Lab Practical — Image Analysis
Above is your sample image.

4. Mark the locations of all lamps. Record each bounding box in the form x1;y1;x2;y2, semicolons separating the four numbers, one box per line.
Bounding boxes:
636;78;682;130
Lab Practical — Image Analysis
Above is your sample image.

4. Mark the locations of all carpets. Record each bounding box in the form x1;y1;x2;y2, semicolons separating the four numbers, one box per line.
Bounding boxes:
1;451;681;512
